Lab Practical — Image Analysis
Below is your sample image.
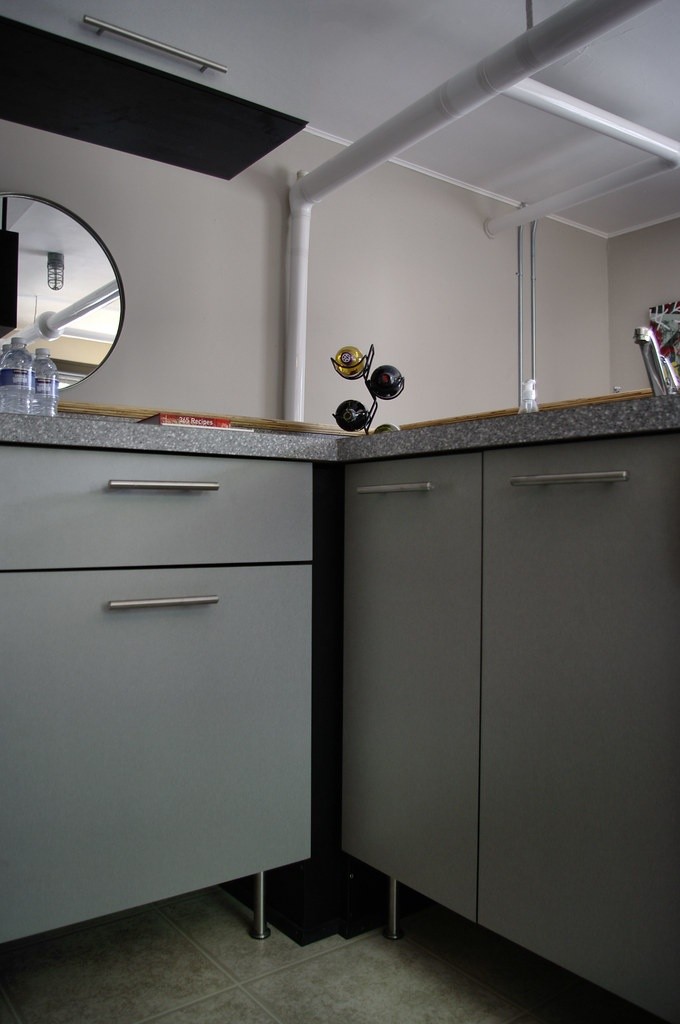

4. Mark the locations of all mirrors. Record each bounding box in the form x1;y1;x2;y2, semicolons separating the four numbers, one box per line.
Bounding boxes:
0;193;126;394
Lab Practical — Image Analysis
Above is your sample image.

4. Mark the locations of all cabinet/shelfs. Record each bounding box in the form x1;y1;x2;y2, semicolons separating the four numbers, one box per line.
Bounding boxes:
323;434;680;1024
0;444;315;942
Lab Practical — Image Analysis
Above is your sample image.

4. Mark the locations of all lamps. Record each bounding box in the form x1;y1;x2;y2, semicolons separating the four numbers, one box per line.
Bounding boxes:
47;252;64;291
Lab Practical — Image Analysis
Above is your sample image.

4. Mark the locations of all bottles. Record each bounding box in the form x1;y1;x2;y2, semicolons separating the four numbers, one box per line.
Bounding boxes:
336;400;367;431
336;346;364;378
374;425;401;433
370;365;403;397
0;344;11;364
29;349;59;417
0;337;32;414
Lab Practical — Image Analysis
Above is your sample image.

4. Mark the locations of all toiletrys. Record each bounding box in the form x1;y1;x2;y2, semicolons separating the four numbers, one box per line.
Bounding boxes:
517;379;537;412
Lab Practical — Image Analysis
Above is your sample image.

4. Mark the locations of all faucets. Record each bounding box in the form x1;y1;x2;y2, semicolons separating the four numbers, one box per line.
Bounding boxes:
633;325;678;395
